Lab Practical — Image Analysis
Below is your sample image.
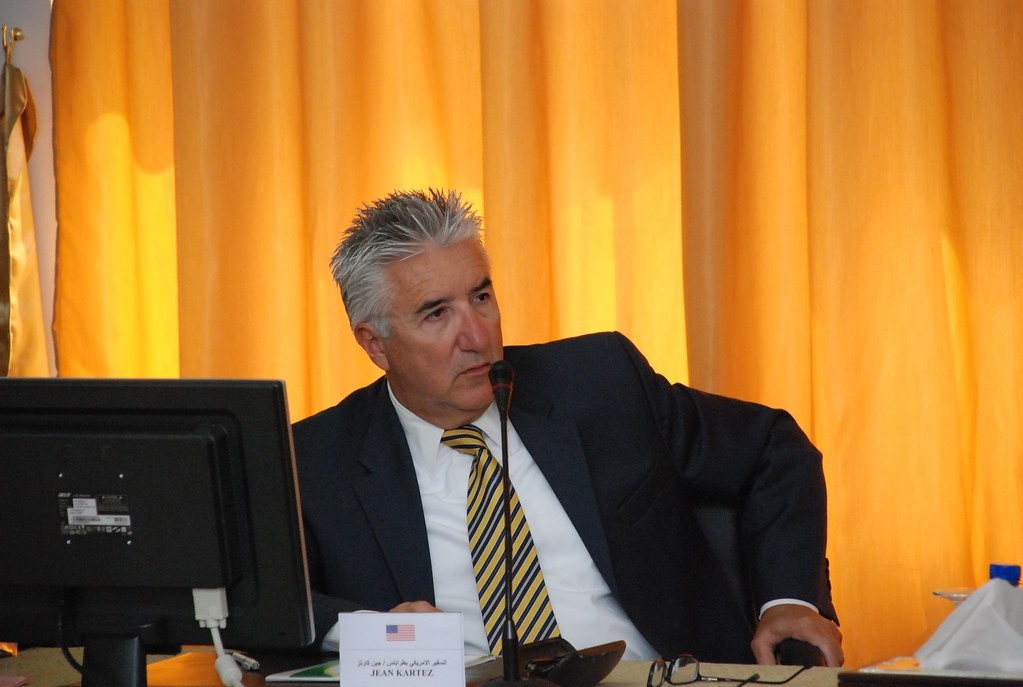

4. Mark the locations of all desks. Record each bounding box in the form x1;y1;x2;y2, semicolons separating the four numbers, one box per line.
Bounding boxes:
2;645;1020;686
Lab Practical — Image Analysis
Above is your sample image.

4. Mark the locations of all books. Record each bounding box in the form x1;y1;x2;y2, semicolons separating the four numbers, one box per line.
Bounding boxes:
264;657;503;684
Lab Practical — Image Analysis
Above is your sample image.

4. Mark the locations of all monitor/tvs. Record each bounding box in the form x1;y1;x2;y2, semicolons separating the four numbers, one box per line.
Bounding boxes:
1;377;316;648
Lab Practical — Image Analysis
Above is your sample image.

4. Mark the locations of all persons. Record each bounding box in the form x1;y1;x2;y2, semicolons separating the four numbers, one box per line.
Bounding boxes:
246;185;846;662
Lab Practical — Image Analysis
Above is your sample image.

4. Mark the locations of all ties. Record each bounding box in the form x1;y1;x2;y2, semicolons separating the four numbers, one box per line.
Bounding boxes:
434;423;574;663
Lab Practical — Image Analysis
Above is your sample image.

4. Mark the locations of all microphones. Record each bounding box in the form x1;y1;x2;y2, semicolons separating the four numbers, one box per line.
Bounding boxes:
478;360;562;687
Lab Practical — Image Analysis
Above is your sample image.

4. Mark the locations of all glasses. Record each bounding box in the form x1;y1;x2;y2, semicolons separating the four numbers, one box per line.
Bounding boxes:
643;653;815;687
520;650;572;678
